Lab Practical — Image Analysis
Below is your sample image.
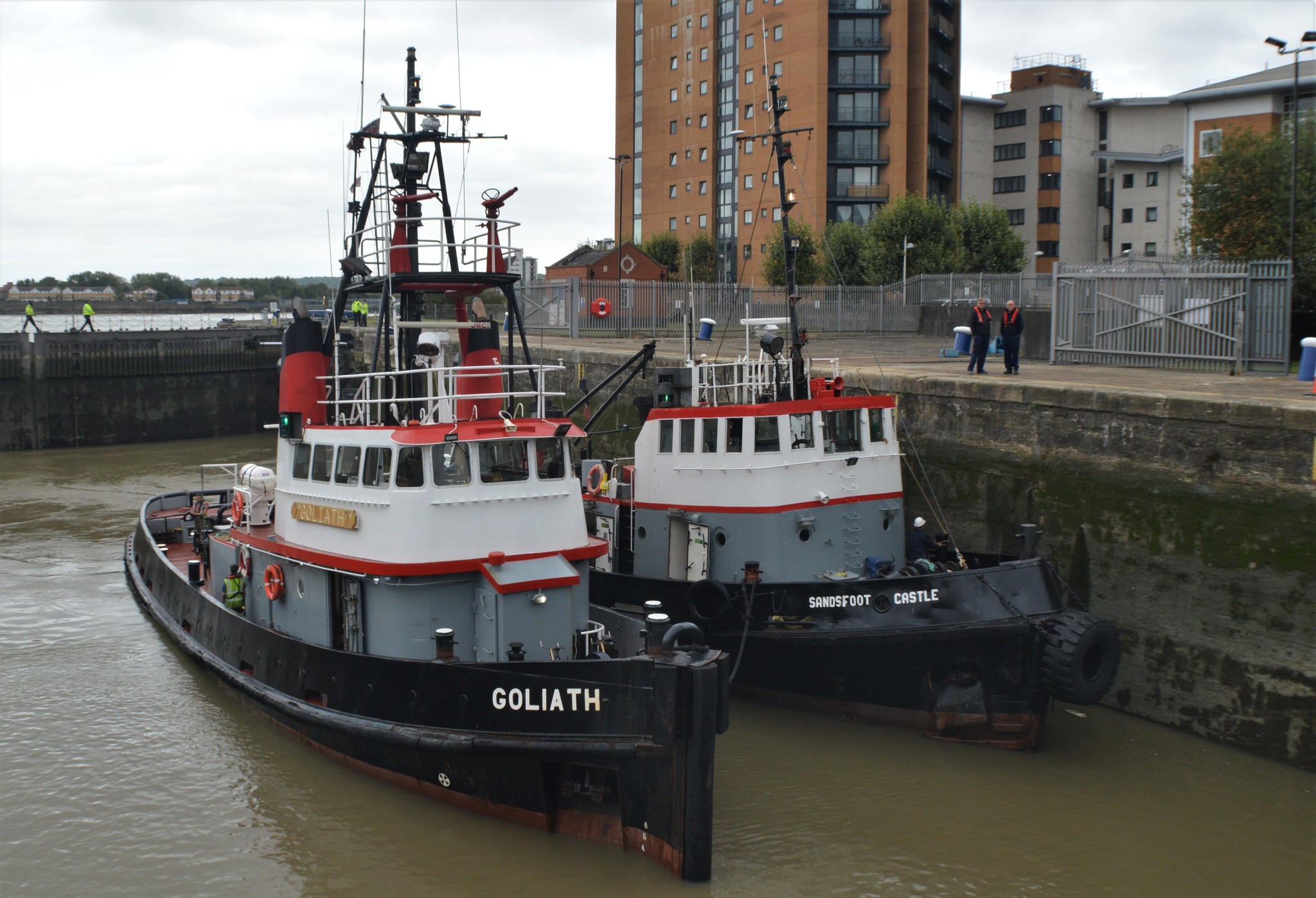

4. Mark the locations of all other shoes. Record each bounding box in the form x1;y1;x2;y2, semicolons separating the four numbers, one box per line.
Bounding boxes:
968;371;972;374
1014;369;1019;374
1004;370;1012;374
977;371;988;374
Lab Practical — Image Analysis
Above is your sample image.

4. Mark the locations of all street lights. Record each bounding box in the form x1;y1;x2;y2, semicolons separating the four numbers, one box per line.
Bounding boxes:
1031;251;1047;275
903;233;916;310
1264;30;1316;266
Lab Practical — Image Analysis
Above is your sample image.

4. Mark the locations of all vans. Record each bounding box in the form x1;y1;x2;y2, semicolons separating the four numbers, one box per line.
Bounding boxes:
926;298;992;307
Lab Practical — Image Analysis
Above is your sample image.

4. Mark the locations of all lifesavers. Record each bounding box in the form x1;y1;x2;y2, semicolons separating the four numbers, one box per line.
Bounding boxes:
231;493;243;526
683;577;731;627
270;563;284;596
264;564;280;601
586;464;605;496
239;545;252;580
1041;609;1122;707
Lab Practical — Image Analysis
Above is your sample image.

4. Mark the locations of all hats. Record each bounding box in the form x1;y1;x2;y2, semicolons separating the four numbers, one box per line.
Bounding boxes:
362;299;366;302
356;297;359;300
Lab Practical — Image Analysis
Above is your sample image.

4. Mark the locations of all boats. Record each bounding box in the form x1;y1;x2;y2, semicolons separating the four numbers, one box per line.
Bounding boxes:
576;17;1122;747
122;5;732;883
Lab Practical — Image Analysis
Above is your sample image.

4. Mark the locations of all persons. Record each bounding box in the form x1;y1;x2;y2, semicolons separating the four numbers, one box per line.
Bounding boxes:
1000;301;1025;375
21;300;43;332
968;297;992;375
352;297;368;327
907;517;941;559
79;300;96;332
191;493;209;551
